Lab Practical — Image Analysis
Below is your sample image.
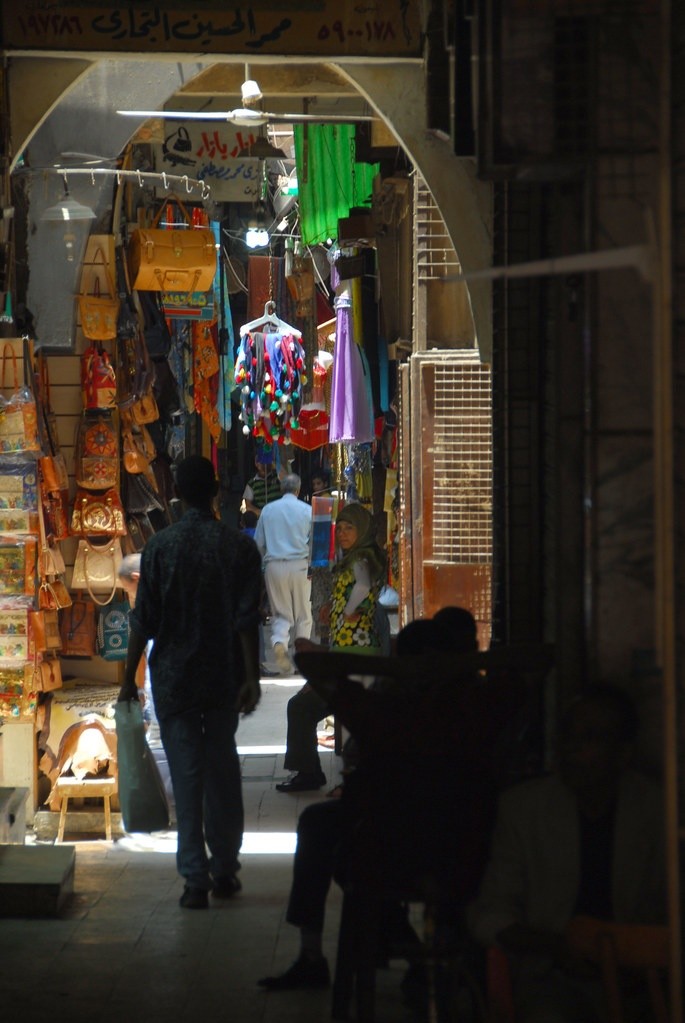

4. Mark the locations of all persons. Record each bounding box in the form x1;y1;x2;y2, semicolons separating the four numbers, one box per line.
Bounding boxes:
251;473;313;671
117;456;261;908
311;470;331;497
276;502;386;789
257;606;669;1023
241;510;258;537
243;454;282;516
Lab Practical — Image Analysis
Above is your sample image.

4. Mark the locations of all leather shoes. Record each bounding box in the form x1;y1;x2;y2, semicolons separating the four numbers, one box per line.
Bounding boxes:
259;664;280;677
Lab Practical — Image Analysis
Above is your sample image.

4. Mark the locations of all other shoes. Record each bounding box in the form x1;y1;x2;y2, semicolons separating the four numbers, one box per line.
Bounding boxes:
276;770;327;792
180;887;209;909
212;875;241;898
258;959;330;990
294;667;301;675
273;643;291;672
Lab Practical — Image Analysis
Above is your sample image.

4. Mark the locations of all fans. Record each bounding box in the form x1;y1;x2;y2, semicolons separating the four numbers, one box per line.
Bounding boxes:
113;62;382;127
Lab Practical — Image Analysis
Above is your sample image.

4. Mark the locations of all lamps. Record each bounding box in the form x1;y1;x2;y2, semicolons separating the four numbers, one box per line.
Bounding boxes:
40;175;97;219
234;128;289;159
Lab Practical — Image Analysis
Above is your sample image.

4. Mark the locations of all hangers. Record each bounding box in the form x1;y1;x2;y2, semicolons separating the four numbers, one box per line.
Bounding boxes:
240;301;302;340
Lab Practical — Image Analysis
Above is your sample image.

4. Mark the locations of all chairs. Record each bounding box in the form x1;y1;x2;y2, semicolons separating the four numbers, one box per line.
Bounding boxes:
331;737;501;1023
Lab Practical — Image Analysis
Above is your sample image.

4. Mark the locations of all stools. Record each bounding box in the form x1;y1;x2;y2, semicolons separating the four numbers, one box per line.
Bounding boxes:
57;776;115;842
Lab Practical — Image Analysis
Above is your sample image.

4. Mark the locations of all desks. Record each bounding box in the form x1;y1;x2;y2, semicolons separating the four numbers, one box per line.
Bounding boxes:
568;915;674;1023
40;678;121;812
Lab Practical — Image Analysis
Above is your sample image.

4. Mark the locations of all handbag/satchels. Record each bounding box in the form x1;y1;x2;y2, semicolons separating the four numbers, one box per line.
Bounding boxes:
112;699;170;833
0;192;218;695
